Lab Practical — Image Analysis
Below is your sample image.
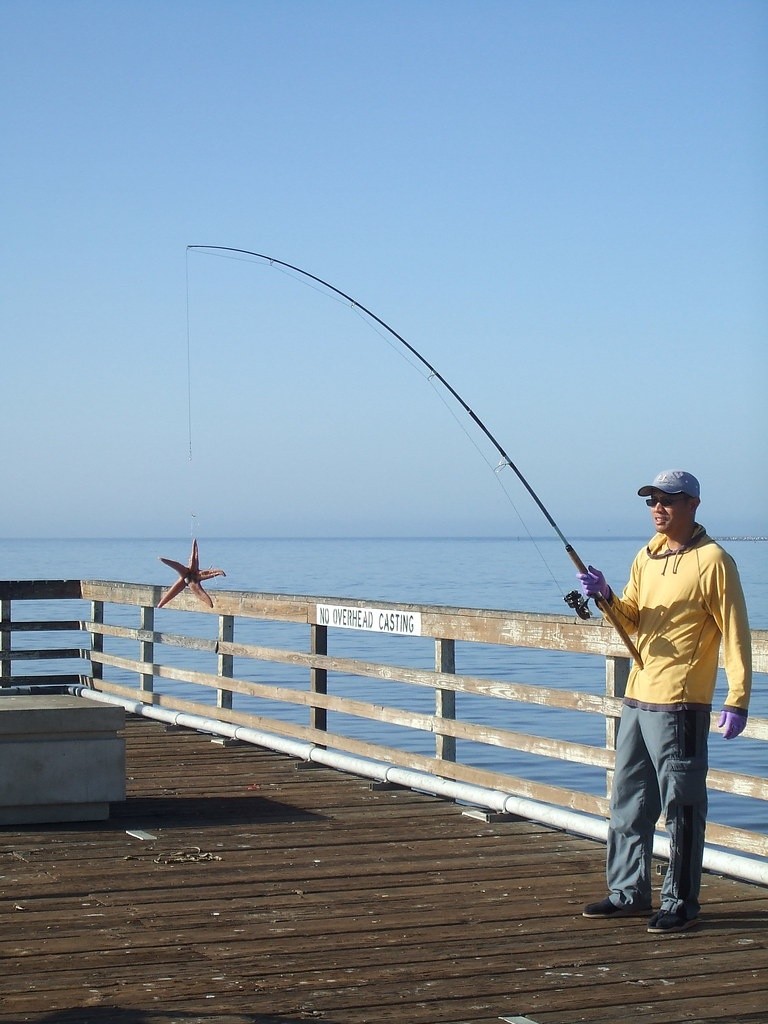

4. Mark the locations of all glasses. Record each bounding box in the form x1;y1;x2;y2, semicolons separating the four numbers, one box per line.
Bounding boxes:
645;496;691;506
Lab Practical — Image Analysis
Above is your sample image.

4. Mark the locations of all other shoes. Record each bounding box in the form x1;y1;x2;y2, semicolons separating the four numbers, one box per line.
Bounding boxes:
646;908;700;933
582;896;654;918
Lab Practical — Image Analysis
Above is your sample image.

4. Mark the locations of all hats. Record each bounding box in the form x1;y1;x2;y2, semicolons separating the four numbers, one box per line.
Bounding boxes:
637;470;700;497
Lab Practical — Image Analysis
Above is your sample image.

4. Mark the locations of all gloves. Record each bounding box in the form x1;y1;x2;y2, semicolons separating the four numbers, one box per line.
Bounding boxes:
575;565;612;602
718;710;748;740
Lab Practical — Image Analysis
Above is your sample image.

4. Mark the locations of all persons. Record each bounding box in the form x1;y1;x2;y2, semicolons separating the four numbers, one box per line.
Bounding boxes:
577;470;754;933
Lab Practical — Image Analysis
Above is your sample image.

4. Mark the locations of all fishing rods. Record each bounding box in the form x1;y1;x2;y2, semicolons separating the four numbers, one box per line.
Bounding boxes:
157;243;646;670
